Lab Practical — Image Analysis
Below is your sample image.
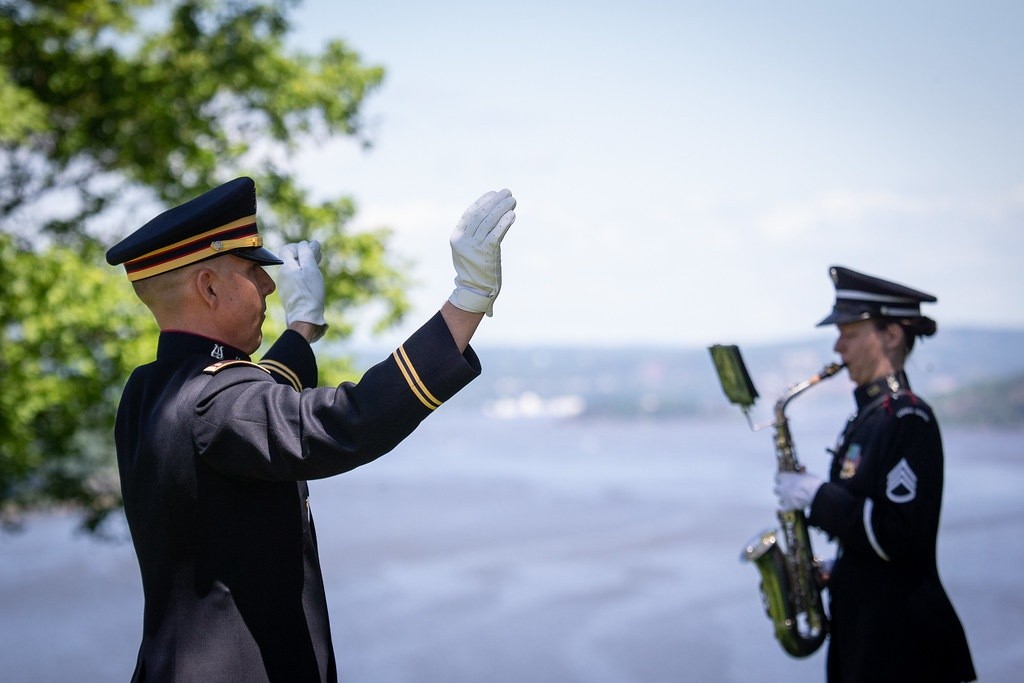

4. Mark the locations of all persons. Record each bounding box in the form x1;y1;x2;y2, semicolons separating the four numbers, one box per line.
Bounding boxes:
775;265;979;683
106;176;518;683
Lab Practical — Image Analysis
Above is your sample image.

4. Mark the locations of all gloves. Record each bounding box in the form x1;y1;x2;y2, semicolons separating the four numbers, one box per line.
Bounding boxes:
773;470;825;511
276;239;329;343
448;189;516;317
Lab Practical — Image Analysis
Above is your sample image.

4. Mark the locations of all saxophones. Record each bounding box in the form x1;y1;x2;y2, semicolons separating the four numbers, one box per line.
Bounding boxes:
739;362;845;658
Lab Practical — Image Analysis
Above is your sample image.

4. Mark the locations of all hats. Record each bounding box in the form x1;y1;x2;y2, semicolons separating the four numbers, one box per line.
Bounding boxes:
106;176;284;283
817;267;937;326
